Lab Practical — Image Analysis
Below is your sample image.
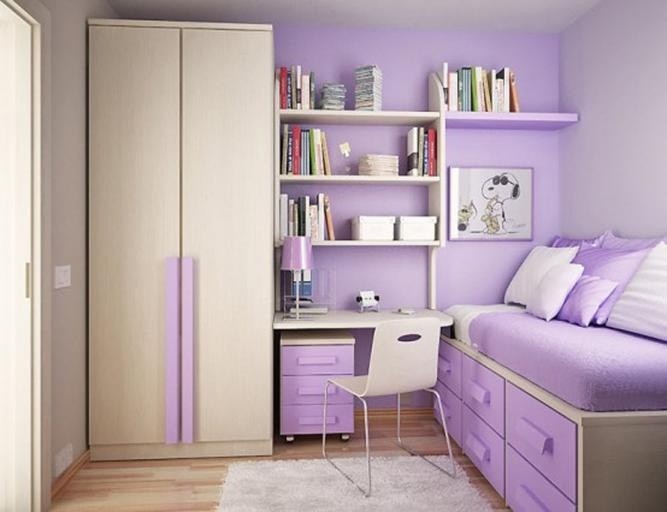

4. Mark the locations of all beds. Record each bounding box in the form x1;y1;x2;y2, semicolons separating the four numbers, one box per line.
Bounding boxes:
443;301;667;411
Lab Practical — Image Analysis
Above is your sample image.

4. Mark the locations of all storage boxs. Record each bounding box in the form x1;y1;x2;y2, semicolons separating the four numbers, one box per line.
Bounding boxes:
348;214;395;243
395;216;437;241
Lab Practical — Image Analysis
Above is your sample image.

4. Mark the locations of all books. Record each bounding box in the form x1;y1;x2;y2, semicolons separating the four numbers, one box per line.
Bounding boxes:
357;153;400;177
293;270;303;295
281;124;335;176
440;61;520;113
274;63;384;112
280;190;335;242
405;127;437;177
303;271;312;296
289;305;330;315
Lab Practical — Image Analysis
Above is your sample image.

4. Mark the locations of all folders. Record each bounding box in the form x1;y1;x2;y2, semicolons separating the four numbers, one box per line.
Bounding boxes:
294;270;312;296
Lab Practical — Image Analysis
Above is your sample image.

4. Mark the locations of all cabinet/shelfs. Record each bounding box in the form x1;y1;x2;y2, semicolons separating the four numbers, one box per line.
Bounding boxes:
83;19;277;463
432;337;461;447
505;381;667;512
280;331;355;442
461;351;505;502
277;72;580;308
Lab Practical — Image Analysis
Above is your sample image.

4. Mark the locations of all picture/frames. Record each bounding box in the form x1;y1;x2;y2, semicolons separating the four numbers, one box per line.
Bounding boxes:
448;166;536;242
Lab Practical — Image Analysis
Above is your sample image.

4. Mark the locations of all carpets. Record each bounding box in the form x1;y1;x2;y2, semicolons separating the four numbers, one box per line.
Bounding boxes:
217;454;493;512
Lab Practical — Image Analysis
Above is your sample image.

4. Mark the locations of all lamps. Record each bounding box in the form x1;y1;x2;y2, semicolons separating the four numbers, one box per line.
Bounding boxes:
280;235;315;321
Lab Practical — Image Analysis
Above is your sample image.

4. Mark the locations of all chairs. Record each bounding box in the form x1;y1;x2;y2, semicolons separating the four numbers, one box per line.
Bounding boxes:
322;317;457;497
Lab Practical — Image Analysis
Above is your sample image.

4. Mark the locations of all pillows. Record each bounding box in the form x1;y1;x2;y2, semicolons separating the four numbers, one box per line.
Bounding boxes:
503;231;667;344
